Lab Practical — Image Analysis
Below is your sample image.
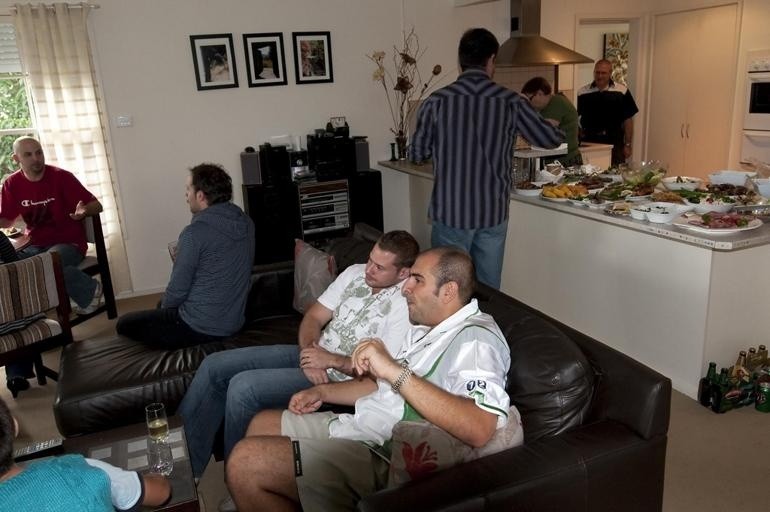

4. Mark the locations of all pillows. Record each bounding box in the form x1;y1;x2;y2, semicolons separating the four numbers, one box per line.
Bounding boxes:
387;404;525;486
293;238;339;316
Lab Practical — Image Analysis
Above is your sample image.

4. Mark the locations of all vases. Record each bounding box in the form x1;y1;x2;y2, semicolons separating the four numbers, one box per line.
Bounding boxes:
395;137;409;160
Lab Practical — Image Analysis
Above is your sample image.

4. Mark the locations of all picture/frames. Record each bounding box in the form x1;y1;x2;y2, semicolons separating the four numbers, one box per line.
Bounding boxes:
291;31;334;84
242;32;288;88
190;33;239;91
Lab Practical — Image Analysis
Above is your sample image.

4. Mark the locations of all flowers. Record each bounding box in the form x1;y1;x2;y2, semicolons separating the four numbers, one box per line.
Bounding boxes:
365;27;443;137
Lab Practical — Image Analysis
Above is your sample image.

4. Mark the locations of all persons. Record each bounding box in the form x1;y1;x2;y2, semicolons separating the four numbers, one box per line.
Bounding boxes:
406;27;567;301
0;395;173;512
0;228;37;398
519;76;582;169
219;243;514;510
178;230;421;485
575;59;639;168
1;134;104;316
116;162;257;351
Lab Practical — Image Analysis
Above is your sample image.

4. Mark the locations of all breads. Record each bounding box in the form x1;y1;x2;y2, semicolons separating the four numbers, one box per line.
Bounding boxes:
541;183;589;199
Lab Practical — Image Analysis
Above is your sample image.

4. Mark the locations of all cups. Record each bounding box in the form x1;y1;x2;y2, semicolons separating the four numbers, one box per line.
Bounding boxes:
146;438;174;476
146;403;169;441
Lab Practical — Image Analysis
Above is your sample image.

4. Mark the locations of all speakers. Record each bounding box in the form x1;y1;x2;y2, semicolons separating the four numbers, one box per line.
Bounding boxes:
307;133;357;181
347;168;385;235
240;182;301;268
355;140;370;172
260;149;291;184
240;151;262;184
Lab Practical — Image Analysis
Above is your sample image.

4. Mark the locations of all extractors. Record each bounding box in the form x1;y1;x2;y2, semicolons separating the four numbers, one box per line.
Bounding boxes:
494;1;594;67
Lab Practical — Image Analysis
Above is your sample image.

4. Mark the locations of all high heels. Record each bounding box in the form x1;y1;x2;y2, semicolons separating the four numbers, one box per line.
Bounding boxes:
7;377;30;398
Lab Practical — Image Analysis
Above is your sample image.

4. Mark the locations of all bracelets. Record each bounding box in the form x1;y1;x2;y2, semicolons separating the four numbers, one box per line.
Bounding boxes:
625;142;631;147
391;366;414;393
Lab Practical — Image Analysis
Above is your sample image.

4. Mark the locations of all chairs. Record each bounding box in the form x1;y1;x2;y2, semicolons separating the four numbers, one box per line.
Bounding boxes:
0;252;73;385
65;215;118;328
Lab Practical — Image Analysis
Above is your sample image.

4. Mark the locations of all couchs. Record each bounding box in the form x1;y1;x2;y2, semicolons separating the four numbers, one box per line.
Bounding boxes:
53;220;672;512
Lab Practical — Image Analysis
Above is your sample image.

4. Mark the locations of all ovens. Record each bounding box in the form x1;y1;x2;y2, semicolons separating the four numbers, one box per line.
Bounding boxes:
742;58;770;130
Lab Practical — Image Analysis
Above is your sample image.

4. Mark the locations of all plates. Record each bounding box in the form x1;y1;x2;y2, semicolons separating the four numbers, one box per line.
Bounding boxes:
516;166;770;235
0;227;21;237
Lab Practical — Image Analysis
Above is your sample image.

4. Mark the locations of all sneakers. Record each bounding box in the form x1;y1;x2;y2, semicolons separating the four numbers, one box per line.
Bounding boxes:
75;281;104;315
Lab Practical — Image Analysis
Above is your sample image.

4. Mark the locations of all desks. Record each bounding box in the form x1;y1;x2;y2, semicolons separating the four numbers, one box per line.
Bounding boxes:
0;229;33;253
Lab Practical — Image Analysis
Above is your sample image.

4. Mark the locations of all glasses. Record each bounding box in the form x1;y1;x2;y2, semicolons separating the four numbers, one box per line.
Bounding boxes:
529;95;534;101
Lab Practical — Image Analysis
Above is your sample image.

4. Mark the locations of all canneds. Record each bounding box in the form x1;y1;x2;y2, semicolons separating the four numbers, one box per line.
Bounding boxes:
755;382;770;413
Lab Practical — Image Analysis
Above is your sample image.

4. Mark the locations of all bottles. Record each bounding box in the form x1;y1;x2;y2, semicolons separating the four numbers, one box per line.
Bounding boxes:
699;343;769;415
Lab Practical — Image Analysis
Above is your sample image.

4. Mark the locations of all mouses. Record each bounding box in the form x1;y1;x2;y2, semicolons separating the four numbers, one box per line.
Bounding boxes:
245;146;255;152
